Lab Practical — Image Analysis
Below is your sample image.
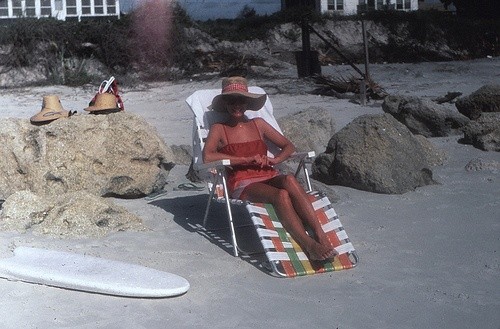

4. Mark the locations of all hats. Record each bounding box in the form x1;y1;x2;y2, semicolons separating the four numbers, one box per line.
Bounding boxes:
211;76;267;111
29;95;77;122
83;92;119;111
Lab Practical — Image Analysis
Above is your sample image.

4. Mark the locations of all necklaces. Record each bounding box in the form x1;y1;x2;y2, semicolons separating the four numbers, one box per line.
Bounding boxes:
229;123;250;129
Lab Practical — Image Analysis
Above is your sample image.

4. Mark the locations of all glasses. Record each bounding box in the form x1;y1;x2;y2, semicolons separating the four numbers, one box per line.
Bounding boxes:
224;97;247;105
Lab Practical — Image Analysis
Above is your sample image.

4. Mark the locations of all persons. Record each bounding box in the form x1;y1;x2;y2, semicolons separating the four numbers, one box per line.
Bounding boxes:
202;77;339;262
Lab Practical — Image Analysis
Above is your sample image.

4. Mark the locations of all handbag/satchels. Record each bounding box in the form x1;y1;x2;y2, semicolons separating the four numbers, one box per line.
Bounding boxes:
90;76;123;111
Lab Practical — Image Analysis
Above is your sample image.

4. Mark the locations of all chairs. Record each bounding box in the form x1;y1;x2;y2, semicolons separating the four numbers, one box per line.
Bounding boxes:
185;86;359;278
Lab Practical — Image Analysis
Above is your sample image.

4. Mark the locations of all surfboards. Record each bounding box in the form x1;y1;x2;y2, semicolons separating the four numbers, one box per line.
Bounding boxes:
1;247;190;298
